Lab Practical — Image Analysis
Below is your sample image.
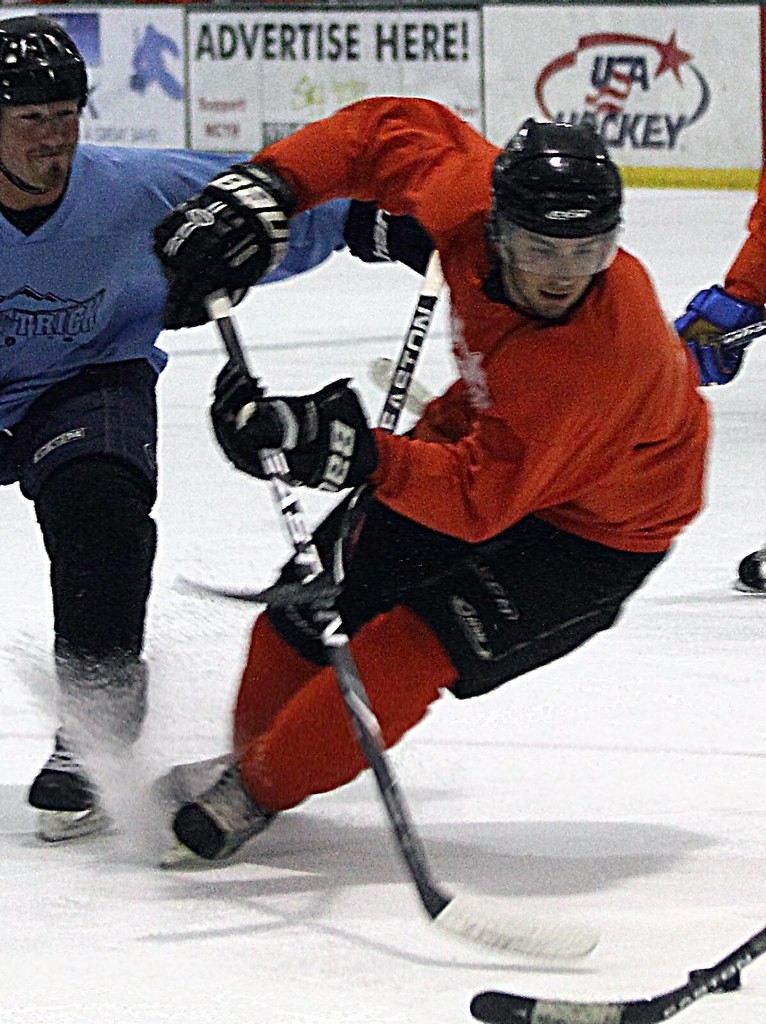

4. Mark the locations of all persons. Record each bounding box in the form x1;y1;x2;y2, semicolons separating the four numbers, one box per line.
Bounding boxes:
147;96;713;865
671;160;766;596
0;14;435;840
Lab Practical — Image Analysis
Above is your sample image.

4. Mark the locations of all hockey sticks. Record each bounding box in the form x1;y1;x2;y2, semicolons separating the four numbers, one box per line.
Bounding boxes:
374;320;765;423
179;245;598;958
172;246;440;606
471;926;766;1023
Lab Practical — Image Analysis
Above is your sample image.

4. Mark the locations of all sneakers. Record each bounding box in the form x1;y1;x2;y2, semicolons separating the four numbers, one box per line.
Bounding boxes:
28;728;115;842
732;547;765;598
161;765;278;869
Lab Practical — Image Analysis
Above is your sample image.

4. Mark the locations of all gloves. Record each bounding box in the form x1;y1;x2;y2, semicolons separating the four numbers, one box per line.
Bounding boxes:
674;284;765;388
344;192;436;276
152;162;295;331
210;359;377;492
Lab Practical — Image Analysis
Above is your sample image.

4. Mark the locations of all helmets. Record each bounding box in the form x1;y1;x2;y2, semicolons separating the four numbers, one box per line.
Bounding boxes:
491;117;623;239
0;16;88;106
